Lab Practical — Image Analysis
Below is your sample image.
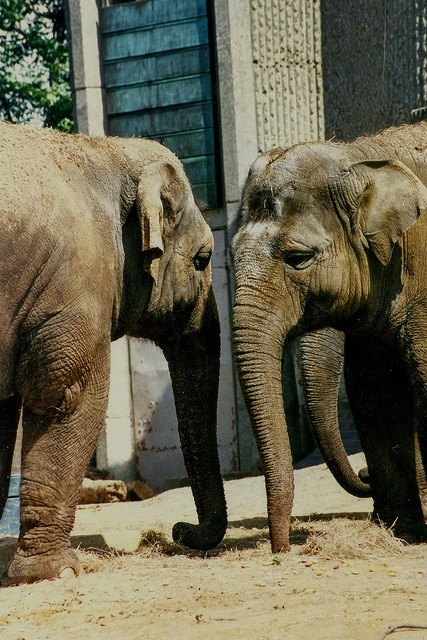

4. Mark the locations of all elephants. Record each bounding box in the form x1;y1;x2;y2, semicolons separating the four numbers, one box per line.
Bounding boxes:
232;122;427;554
0;121;228;582
293;328;373;497
